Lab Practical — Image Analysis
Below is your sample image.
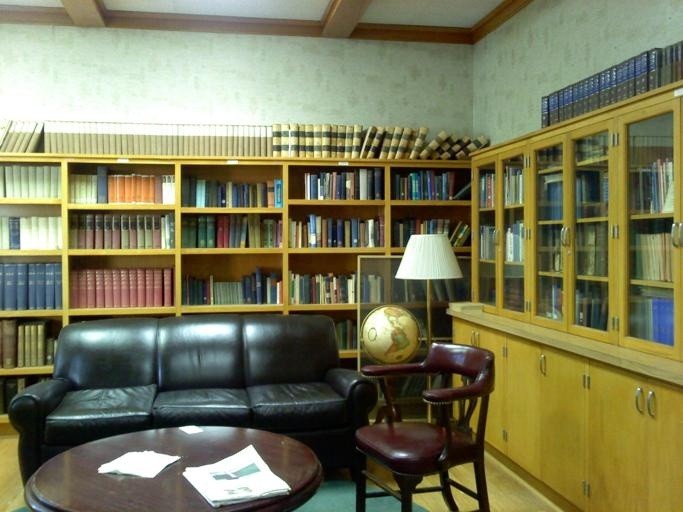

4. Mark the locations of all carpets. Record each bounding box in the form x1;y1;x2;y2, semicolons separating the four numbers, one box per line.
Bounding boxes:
11;478;430;512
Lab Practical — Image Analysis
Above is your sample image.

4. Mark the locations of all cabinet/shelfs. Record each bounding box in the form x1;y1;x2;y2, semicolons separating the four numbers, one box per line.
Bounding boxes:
447;81;683;512
0;154;471;424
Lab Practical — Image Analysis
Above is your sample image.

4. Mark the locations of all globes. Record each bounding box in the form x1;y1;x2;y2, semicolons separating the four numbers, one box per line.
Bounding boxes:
360;304;423;425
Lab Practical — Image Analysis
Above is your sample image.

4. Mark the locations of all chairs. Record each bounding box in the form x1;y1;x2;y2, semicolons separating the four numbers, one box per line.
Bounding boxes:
352;343;495;512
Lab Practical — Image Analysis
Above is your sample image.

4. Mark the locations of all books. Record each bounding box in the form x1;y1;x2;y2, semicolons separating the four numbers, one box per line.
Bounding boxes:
288;168;385;397
392;171;471;398
0;164;61;413
0;120;489;159
479;174;496;299
538;144;562;318
68;175;175;319
540;41;682;126
576;135;609;329
182;178;283;304
504;165;523;311
631;136;674;346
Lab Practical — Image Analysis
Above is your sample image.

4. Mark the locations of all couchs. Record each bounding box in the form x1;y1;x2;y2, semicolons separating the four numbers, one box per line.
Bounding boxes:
8;314;377;487
